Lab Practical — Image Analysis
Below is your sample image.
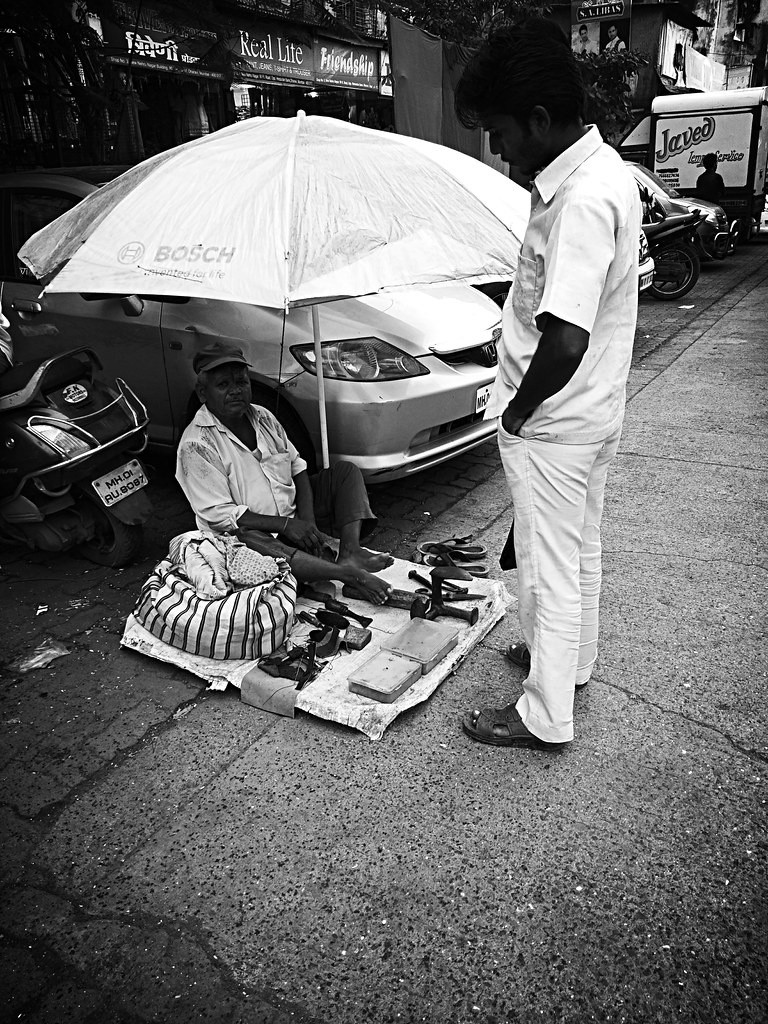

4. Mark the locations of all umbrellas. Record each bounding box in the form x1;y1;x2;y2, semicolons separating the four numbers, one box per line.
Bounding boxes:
18;108;531;469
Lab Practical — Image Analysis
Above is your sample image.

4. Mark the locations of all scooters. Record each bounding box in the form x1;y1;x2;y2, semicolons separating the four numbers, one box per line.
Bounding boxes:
0;313;155;566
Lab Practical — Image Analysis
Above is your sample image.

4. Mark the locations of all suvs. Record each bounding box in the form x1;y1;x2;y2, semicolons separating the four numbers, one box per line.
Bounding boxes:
623;161;742;260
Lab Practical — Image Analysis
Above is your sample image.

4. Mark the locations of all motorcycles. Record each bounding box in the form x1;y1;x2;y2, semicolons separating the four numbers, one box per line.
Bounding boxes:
642;208;709;301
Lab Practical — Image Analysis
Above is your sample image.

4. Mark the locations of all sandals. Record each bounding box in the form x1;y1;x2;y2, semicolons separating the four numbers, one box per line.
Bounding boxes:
507;643;587;689
462;700;564;752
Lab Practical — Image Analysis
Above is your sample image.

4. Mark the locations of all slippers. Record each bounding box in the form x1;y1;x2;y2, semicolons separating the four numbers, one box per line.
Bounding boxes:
417;539;487;559
423;550;490;577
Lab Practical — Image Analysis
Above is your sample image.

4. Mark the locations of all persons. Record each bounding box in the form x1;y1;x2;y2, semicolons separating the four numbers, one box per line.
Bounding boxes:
574;24;597;55
605;24;626;54
695;154;727;206
454;20;642;749
175;342;394;607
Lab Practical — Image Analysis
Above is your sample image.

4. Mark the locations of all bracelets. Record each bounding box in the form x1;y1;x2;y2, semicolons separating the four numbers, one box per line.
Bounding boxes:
282;517;289;533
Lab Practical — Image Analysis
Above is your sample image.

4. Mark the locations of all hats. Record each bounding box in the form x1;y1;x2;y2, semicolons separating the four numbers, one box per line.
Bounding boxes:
193;341;253;376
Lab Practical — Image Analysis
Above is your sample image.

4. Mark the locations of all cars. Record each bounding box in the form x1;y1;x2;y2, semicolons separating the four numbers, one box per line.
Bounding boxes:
0;167;503;487
479;229;654;312
